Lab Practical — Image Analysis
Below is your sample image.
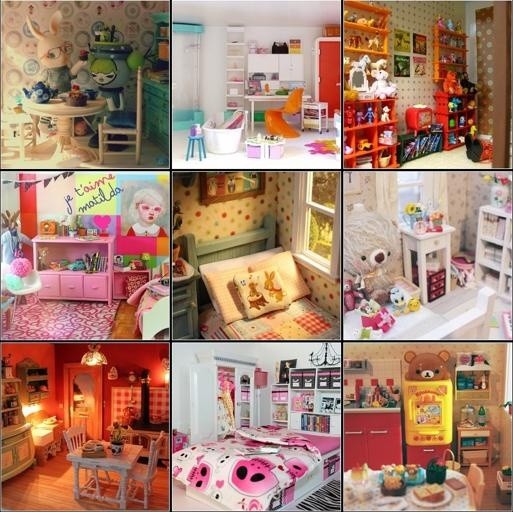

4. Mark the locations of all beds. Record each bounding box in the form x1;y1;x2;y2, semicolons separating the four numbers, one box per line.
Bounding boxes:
136;273;169;340
184;213;340;339
172;429;340;511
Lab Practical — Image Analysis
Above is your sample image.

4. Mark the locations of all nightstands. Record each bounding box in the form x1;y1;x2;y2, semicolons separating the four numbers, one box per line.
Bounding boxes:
174;256;202;340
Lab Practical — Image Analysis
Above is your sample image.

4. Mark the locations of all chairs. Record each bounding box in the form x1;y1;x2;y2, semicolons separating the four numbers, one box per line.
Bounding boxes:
115;430;165;509
468;463;485;510
98;65;142;166
5;269;44;325
62;424;110;497
1;112;36;164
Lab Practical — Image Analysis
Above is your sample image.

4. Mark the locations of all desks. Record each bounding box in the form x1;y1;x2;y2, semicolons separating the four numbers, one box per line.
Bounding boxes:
22;95;105;166
244;92;312;133
66;441;143;509
400;223;456;305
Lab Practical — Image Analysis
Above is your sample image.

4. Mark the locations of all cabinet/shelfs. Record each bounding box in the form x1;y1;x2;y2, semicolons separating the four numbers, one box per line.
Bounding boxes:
343;408;404;472
151;11;170;70
288;367;341;434
344;98;401;168
0;378;36;483
271;384;288;426
433;24;470;84
344;0;393;99
143;79;169;156
225;26;246;112
457;421;495;468
113;266;152;303
31;233;116;305
474;205;513;298
455;364;492;402
248;54;305;95
433;91;477;151
16;367;49;405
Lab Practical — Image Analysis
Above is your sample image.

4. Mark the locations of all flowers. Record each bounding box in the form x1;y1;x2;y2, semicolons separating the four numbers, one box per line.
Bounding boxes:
478;171;513;185
112;421;122;443
403;202;444;221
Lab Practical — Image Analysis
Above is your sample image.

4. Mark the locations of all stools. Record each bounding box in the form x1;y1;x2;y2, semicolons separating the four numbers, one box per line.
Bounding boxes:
301;102;329;134
186;135;206;161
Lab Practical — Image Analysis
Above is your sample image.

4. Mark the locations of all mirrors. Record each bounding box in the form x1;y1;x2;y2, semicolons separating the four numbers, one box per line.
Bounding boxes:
235;367;255;429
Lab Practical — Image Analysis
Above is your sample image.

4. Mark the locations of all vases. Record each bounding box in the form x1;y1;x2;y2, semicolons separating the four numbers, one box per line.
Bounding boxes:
490;184;508;208
111;442;124;456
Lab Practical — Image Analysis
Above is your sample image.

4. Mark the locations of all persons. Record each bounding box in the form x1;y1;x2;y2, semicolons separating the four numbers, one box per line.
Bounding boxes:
114;256;123;266
126;188;168;237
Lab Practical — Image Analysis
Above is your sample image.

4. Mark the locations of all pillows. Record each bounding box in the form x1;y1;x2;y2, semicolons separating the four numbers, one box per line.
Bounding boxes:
106;110;136;127
199;246;312;324
162;262;170;278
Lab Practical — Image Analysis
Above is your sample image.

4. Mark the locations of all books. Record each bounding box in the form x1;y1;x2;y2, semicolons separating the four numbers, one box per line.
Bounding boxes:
403;134;442;162
482;214;506;261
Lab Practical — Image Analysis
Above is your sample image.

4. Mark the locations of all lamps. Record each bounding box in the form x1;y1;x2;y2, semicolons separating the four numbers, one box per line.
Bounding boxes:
81;343;108;366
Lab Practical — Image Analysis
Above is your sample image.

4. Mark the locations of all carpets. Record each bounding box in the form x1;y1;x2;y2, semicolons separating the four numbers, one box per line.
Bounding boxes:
1;299;122;340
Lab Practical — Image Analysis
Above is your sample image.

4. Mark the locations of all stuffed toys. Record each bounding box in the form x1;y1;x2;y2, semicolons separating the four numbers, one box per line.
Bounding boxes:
344;10;387;72
343;103;396;155
438;16;465;64
443;70;476;96
370;70;398;100
343;212;420;331
448;98;478;146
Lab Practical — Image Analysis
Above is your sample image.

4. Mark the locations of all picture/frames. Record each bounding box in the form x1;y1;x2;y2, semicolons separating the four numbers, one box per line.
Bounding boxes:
199;172;265;205
279;359;297;384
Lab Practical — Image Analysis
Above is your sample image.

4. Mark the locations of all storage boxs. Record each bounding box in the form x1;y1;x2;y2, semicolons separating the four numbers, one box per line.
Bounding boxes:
496;471;512;504
245;136;286;160
